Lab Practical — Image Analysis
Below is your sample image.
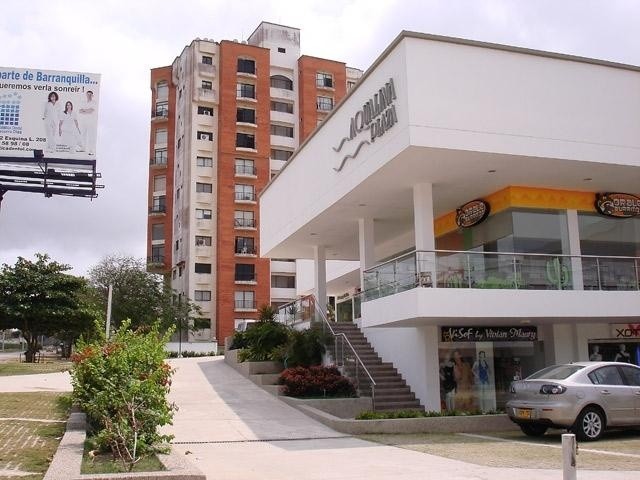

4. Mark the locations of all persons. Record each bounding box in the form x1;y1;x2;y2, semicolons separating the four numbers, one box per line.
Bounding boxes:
439;350;458;415
41;90;62;154
588;343;603;361
470;350;490;416
58;100;82;155
75;90;95;156
451;349;473;411
612;344;630;364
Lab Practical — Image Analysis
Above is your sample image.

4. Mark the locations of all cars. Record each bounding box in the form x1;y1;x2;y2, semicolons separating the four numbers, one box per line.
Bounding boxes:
505;361;640;442
